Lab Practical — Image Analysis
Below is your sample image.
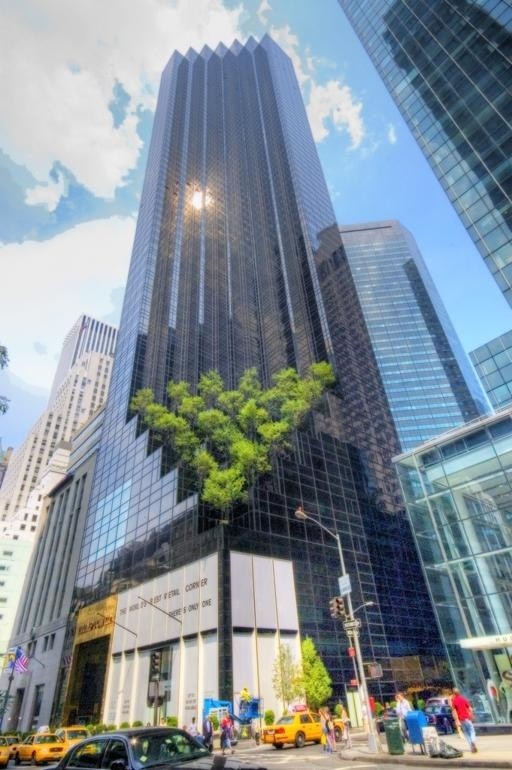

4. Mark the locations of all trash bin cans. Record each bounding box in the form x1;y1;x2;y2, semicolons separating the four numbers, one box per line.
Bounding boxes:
383;717;405;755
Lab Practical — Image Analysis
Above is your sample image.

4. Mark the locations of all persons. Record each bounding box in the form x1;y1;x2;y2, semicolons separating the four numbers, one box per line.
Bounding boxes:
483;672;502;723
317;703;354;753
188;712;255;756
383;690;426;746
499;671;512;723
449;686;478;752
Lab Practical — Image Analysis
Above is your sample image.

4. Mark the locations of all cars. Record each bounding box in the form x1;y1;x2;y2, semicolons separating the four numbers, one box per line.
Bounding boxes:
376;690;490;732
0;725;97;769
41;725;268;770
260;710;345;750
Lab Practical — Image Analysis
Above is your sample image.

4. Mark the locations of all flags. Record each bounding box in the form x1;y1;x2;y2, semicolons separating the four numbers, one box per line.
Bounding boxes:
15;647;30;673
7;647;18;674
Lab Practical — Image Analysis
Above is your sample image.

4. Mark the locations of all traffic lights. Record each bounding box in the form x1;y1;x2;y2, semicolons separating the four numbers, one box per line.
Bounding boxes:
329;595;346;620
151;650;162;673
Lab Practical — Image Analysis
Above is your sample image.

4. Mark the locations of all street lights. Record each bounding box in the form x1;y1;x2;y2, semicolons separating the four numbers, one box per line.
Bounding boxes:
294;508;383;755
345;599;374;734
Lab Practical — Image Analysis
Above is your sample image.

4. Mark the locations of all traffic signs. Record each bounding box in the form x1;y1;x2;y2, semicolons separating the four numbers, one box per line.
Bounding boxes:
342;618;362;638
